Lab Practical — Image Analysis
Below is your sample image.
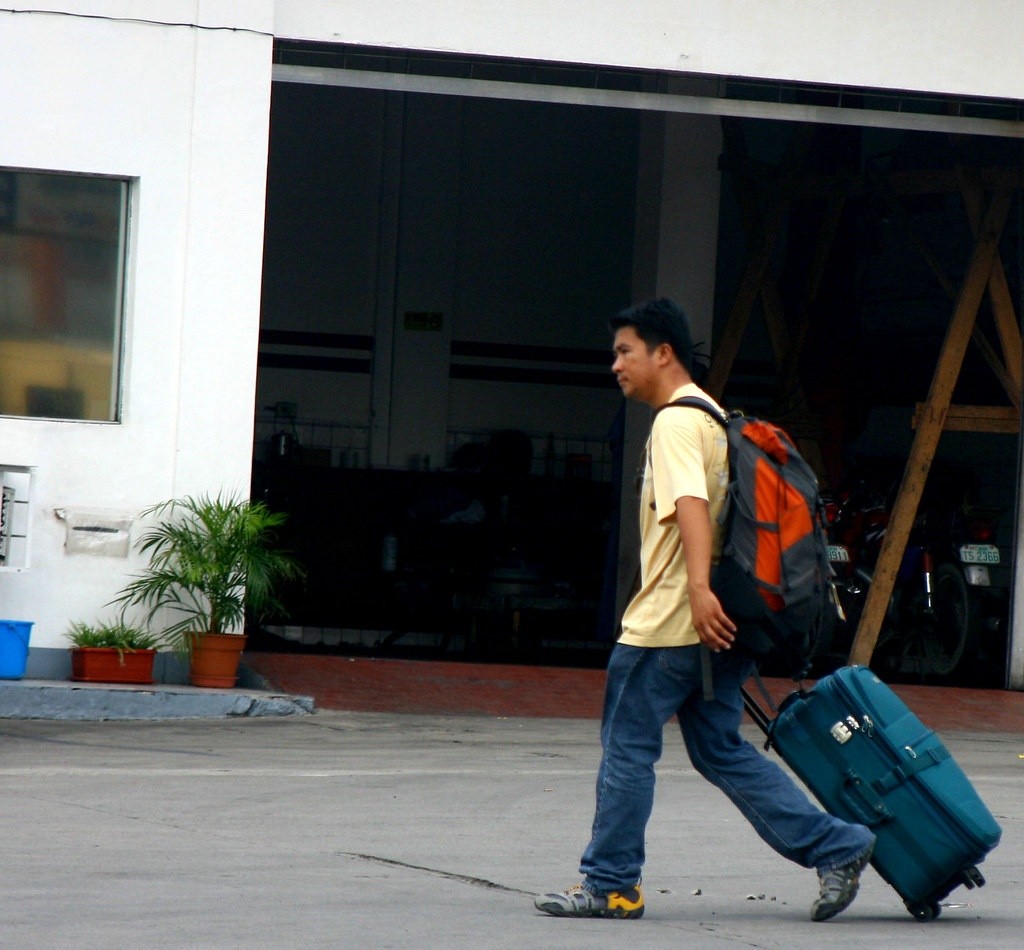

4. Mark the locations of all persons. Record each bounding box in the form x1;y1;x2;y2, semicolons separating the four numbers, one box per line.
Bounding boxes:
533;307;875;921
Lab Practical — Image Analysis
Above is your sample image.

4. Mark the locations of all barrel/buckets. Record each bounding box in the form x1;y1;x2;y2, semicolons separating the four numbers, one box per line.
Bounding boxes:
0;619;35;679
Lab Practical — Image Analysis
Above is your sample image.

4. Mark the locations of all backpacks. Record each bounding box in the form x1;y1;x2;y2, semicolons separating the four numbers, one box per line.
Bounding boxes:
652;396;845;680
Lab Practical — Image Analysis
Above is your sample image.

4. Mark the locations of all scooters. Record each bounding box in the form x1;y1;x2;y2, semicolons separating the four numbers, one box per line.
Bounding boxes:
798;483;1002;680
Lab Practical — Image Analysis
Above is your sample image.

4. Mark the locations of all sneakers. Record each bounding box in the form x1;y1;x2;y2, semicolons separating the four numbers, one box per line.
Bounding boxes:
811;842;871;921
534;884;645;921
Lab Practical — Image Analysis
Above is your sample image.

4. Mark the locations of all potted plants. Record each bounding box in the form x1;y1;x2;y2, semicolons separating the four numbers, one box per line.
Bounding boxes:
68;619;162;685
100;496;300;689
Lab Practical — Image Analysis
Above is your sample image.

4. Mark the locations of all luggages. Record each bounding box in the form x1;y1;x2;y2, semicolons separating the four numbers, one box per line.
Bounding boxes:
740;665;1002;921
